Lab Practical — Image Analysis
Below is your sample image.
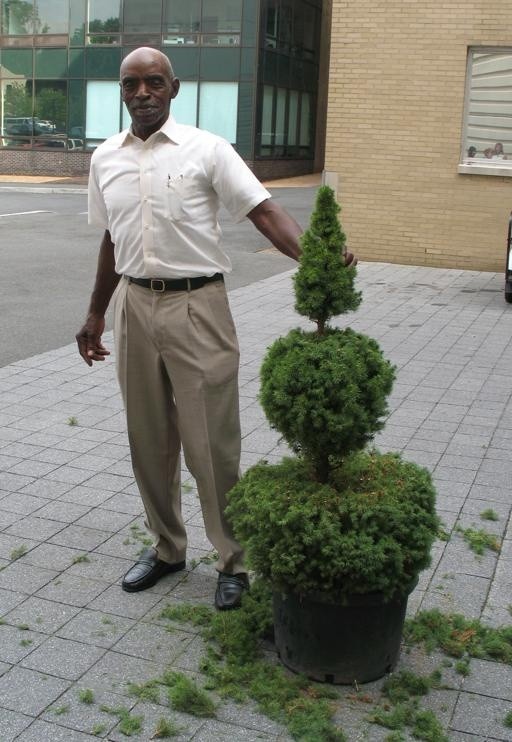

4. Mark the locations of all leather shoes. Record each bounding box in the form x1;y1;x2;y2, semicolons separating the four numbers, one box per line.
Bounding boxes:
214;571;251;611
122;546;186;592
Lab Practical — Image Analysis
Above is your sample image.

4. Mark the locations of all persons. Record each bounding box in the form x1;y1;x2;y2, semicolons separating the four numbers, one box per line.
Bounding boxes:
77;42;357;610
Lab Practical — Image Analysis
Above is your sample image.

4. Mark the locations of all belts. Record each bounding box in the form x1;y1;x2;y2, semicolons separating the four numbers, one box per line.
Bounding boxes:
124;273;224;293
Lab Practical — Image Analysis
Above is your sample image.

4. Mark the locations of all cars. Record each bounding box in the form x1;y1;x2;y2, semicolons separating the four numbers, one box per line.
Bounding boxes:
1;118;83;152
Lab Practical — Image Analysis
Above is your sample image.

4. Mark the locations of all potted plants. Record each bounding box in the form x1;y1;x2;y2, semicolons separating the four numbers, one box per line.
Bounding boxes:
223;185;442;683
